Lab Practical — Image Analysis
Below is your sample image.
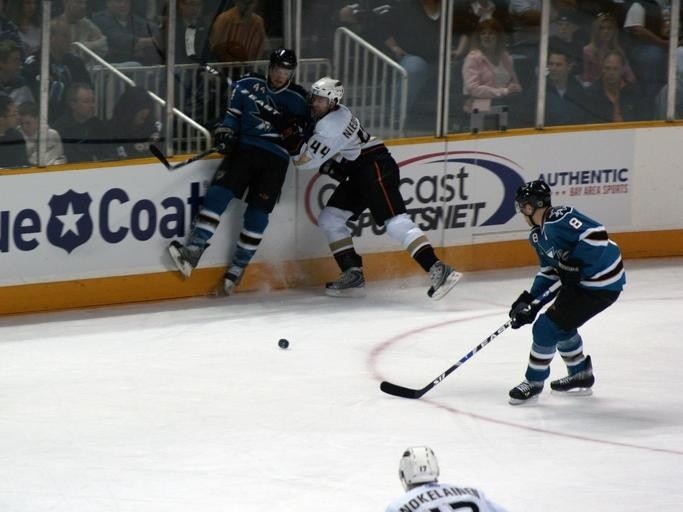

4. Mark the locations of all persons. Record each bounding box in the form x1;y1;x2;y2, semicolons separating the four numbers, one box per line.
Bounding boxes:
508;178;627;401
167;46;317;299
282;76;455;298
384;444;512;512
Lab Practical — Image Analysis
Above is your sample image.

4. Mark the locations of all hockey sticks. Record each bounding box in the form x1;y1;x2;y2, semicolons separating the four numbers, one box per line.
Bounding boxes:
149;143;226;171
380;281;563;399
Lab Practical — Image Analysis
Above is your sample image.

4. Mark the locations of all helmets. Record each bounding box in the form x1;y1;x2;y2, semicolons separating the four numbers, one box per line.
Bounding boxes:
512;181;552;211
268;47;297;71
398;445;440;486
310;76;344;106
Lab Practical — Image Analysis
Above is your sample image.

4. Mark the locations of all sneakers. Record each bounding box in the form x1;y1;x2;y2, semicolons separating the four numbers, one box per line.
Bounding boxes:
325;267;366;290
550;354;596;390
508;376;546;400
170;238;211;269
426;262;455;297
223;260;249;285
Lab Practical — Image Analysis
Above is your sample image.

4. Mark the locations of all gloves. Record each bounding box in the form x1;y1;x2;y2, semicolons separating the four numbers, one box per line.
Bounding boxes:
510;290;541;330
320;159;361;183
212;123;235;154
